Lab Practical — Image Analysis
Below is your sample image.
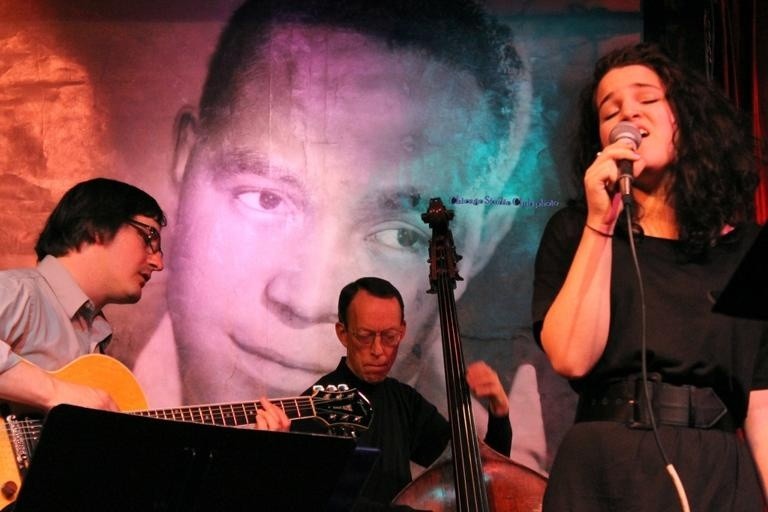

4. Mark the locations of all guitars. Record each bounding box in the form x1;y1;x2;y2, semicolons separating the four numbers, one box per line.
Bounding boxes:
0;354;377;511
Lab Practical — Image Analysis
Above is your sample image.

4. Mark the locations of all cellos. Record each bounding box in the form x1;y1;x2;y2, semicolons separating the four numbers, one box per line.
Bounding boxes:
392;197;549;511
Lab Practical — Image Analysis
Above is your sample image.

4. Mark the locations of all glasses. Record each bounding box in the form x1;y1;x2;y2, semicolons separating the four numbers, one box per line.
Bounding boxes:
340;325;406;350
122;217;163;254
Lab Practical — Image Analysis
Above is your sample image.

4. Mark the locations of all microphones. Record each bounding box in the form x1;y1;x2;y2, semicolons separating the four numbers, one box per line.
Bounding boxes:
609;120;642;207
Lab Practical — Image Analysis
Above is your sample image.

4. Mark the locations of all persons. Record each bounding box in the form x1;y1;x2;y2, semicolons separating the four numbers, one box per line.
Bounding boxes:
530;37;768;512
286;277;512;512
130;2;549;480
1;178;292;512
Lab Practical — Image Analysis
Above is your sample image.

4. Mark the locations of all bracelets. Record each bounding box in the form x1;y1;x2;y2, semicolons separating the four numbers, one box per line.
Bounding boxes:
585;223;614;238
